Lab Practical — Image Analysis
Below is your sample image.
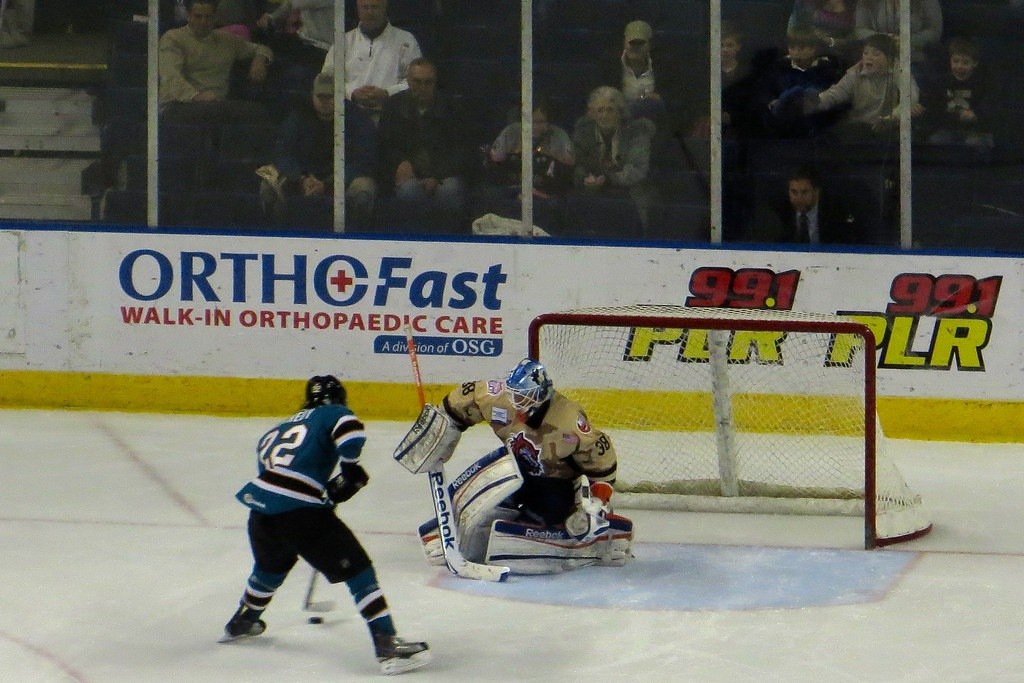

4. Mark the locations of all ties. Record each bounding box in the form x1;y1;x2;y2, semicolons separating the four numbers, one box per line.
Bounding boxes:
798;214;809;246
599;128;616;163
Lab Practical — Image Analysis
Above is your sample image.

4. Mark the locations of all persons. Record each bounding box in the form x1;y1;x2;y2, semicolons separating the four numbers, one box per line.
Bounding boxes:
803;35;920;130
380;57;466;222
786;0;942;46
158;0;335;121
318;0;422;102
216;375;432;674
393;357;632;582
921;36;980;124
490;84;650;186
261;74;376;233
774;163;860;244
720;19;741;124
591;20;671;96
772;28;832;95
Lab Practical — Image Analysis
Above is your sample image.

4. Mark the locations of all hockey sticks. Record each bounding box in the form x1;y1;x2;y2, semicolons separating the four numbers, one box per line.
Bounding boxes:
404;323;510;582
304;569;333;613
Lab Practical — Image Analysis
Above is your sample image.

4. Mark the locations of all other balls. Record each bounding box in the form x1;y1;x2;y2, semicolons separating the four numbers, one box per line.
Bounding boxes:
308;617;321;624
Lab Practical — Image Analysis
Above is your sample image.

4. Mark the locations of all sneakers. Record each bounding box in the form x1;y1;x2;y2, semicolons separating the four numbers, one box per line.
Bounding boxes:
218;607;267;643
368;622;432;676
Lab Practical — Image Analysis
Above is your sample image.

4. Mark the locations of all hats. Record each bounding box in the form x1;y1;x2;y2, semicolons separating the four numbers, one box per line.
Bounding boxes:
624;21;652;44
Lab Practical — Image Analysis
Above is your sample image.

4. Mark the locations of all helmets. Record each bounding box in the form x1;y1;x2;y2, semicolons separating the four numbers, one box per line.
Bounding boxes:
505;358;553;415
299;375;346;412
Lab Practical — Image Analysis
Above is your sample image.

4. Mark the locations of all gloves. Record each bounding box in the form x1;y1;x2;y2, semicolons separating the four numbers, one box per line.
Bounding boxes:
328;462;369;501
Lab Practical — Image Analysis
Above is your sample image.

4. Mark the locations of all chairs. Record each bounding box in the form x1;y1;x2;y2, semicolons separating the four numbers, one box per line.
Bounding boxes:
94;0;1024;256
